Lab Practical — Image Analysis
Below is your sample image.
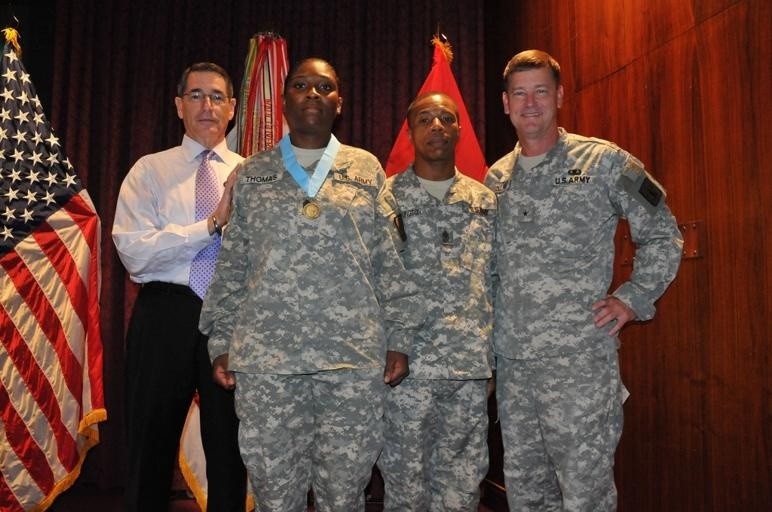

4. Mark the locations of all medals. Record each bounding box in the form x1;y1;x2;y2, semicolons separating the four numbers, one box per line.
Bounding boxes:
303;202;321;219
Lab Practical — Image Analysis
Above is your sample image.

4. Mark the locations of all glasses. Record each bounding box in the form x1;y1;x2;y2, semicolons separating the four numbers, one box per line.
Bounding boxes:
183;91;231;104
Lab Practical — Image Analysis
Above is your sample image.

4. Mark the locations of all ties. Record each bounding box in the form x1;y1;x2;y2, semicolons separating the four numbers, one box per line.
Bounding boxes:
189;150;221;301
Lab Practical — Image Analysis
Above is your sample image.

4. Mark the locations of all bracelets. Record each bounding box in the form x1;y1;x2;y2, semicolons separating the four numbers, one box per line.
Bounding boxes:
211;209;222;236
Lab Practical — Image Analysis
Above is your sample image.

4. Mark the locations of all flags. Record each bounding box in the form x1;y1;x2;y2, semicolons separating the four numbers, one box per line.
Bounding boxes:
0;28;107;512
386;37;488;180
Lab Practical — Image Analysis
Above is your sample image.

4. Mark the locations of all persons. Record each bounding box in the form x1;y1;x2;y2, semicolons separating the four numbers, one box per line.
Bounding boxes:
483;49;686;512
377;91;499;510
196;58;427;509
110;63;248;511
